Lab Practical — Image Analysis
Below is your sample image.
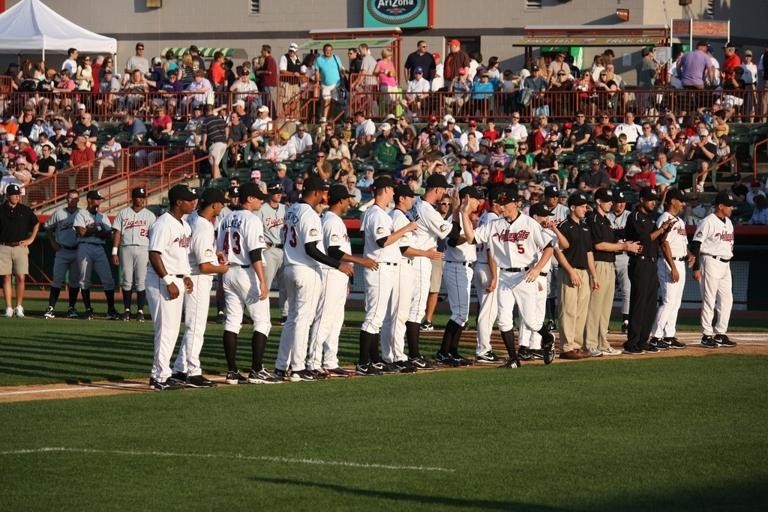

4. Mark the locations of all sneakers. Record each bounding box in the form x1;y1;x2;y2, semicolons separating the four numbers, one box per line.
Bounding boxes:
542;332;556;364
644;342;661;354
274;368;292;381
226;370;248;384
586;348;603;357
248;363;282;384
66;306;80;317
649;339;669;350
169;371;187;383
15;304;24;318
310;368;330;379
185;374;213;388
148;377;184;391
713;334;736;347
372;360;397;373
85;307;95;317
476;351;503;364
393;362;417;373
136;309;145;322
622;346;645;355
123;308;131;322
105;310;121;321
435;351;466;366
355;362;384;376
560;350;583;359
498;358;521;370
662;337;686;349
290;370;316;381
700;334;719;348
601;346;622;356
407;356;435;370
419;320;435;331
327;368;349;377
44;305;56;319
696;185;704;193
5;306;14;318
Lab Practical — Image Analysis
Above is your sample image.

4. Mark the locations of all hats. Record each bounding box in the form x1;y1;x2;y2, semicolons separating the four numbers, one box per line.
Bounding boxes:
5;184;22;196
750;179;761;187
19;136;30;144
567;192;588;205
327;185;356;205
2;152;10;158
78;104;85;110
493;160;504;168
458;67;465;76
548;168;560;174
731;172;741;180
167;184;198;202
402;154;413;165
715;191;738;206
366;166;375;172
722;42;734;48
447;117;456;124
699;129;709;137
448;39;460;46
753;194;768;208
639;157;649;166
530;64;539;71
104;133;115;141
199;187;231;206
6;134;15;141
544;184;560;195
238;182;270;200
393;184;421;198
744;50;752;57
267;182;284;194
16;158;25;164
558;189;568;198
319;117;327;122
529;202;555;216
454;169;462;177
383;113;398;124
287;43;299;52
339;169;348;176
428;115;437;121
6;161;16;168
258;105;269;112
414;66;423;73
562;122;572;129
66;130;76;137
665;188;688;202
250;169;262;178
468;120;476;127
430;137;439;145
378;123;391;130
479;139;490;147
602;152;615;160
594;188;615;201
717;134;728;140
639;186;658;200
426;174;454;189
371;176;397;189
458;185;481;200
280;131;289;140
274;162;287;170
494;191;519;204
230;186;240;197
132;187;147;198
612;190;626;202
302;176;324;191
87;190;105;200
232;100;245;109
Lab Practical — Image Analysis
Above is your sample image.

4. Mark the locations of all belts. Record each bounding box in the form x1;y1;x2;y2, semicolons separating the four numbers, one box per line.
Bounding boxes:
673;256;685;262
462;261;473;268
499;266;530;273
1;241;20;247
712;255;730;263
637;254;657;263
540;271;549;277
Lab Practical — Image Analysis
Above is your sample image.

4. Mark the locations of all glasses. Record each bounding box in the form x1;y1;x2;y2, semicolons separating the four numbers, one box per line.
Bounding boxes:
600;73;606;77
549;194;559;197
513;116;520;119
460;163;467;166
298;128;305;131
680;138;685;140
290;46;297;49
605;116;608;119
437;202;452;206
557;72;566;76
582;73;589;77
521;148;526;150
592;162;600;166
53;118;60;121
539;117;545;120
577;115;584;118
316;155;325;158
482;172;489;175
422;46;426;48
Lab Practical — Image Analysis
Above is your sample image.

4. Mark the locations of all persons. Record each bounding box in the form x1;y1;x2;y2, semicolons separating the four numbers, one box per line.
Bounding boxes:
50;116;71;137
74;54;95;114
424;116;439;137
67;135;94;187
215;103;231;139
358;163;374;188
248;168;267;195
119;112;148;146
579;157;608;192
687;193;704;226
319;124;339;152
163;48;181;82
230;67;259;110
546;51;571;82
517;203;569;361
272;131;296;161
421;135;444;161
14;157;33;183
442;67;471;115
747;178;766;205
51;69;75;114
116;70;149;112
655;129;676;152
112;187;156;322
705;44;720;89
32;60;46;80
684;115;700;137
183;123;203;148
208;51;225;92
571;111;592;152
718;135;733;164
397;114;417;137
351;134;371;161
541;185;570;225
533;142;558;172
148;105;172;141
123;41;150;75
18;105;36;137
463;132;479;153
313;44;347;122
493;112;527;144
287;177;305;203
326;136;350;161
28;116;53;139
516;64;530;91
618;134;632;157
229;112;247;165
270;163;293;198
548;120;562;141
400;126;416;154
445;39;471;80
562;166;581;193
499;128;518;156
352;43;378;89
713;111;730;136
240;60;256;82
602;49;616;62
665;50;685;89
59;135;76;170
189;45;205;72
557;122;576;155
265;138;278;161
60;105;73;119
90;54;104;93
397;155;423;183
181;104;202;131
405;66;430;103
372;132;406;166
748;194;767;225
490;168;518;197
223;59;237;97
689;131;718;194
14;61;32;83
623;186;677;354
442;118;461;137
595;70;618;96
173;73;214;118
383;185;446;374
300;54;315;77
676;42;714;89
592;55;605;83
635;48;656;87
592;111;616;136
613;112;644;145
178;50;193;80
459;121;483;145
727;170;748;193
289;123;310;152
2;133;19;152
433;50;444;92
690;190;737;347
96;69;121;113
605;62;624;94
649;188;695;350
412;128;431;152
97;58;113;92
602;153;625;187
67;113;99;142
554;192;601;359
630;158;656;190
0;186;40;319
501;69;516;89
624;151;644;184
659;111;682;136
20;69;42;93
548;131;561;154
542;169;561;191
511;155;538;182
274;178;353;381
373;48;399;117
279;42;301;120
145;184;199;392
484;119;501;144
436;194;451;219
667;132;688;166
487;57;501;91
523;191;540;216
141;55;164;90
636;122;659;154
344;175;362;215
32;132;57;163
403;171;425;198
257;182;289;322
2;61;19;81
170;188;228;388
422;153;451;177
730;188;752;226
374;123;394;142
227;187;241;211
741;49;758;123
444;170;470;193
666;124;676;142
584;188;643;356
3;162;24;196
256;45;278;115
76;102;85;114
250;104;272;161
510;144;538;170
224;177;240;203
593;126;619;153
521;181;544;197
216;187;240;320
491;141;512;171
406;174;459;369
471;160;482;182
218;184;283;385
94;134;121;181
145;70;183;117
0;109;19;136
472;138;490;165
559;191;568;205
435;187;479;367
201;103;230;178
492;160;505;183
17;67;59;124
527;120;546;149
16;137;35;162
186;59;205;81
135;128;170;169
309;150;332;180
470;73;493;99
441;130;463;155
516;196;525;216
337;118;354;142
332;157;356;186
30;145;56;177
446;158;473;184
16;152;33;171
60;47;78;80
353;110;376;142
0;154;9;174
72;190;121;320
44;190;80;318
534;55;547;80
252;54;265;71
311;117;327;150
720;42;741;71
471;168;492;197
475;189;507;364
380;113;401;140
719;66;746;91
340;48;361;75
459;188;553;370
685;122;710;144
535;113;550;139
655;182;670;213
318;125;333;153
523;65;546;98
233;98;251;131
440;142;461;167
246;135;265;161
546;70;572;92
354;177;417;376
559;160;574;180
758;42;768;122
404;41;436;80
572;71;597;98
51;126;63;145
305;185;379;380
651;153;679;213
